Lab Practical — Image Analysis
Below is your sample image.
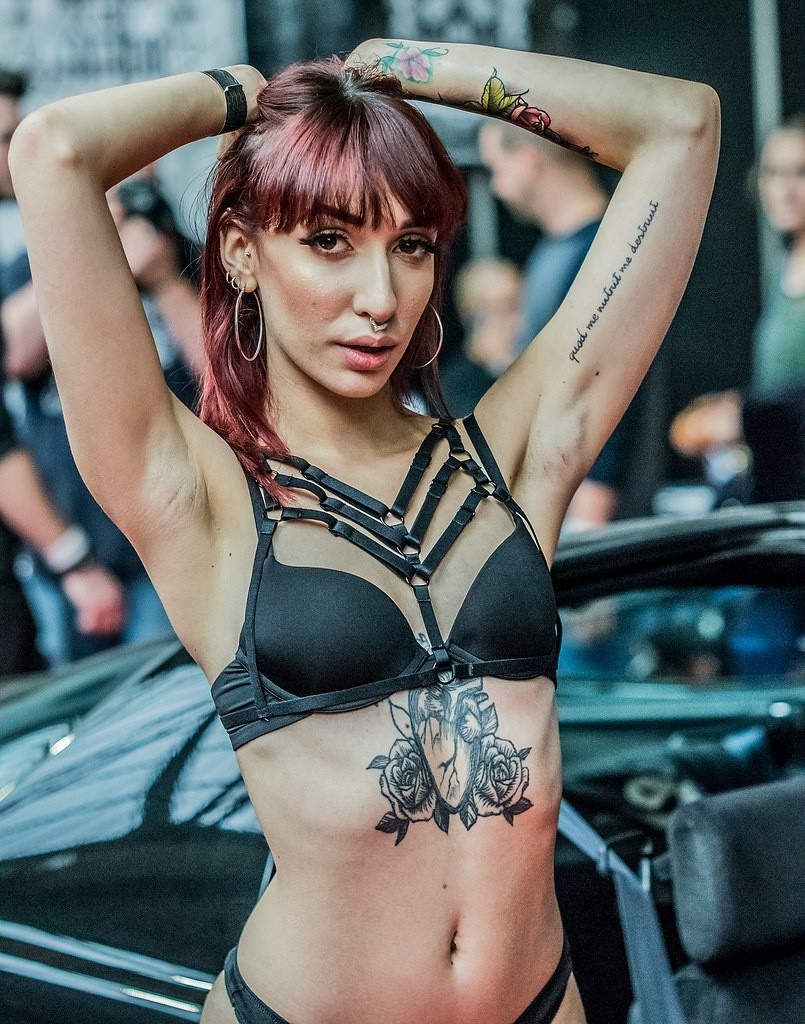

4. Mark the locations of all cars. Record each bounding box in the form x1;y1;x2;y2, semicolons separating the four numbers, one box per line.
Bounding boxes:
0;500;804;1024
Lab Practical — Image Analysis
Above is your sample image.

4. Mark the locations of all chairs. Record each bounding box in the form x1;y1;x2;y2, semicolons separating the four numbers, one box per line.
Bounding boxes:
627;779;805;1024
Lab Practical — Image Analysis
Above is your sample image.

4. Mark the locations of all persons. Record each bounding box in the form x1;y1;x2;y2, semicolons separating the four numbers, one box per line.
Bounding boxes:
0;69;623;677
7;36;722;1024
671;116;805;496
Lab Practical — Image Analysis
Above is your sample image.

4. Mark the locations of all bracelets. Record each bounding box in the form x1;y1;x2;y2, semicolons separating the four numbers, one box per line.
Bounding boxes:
199;68;247;137
